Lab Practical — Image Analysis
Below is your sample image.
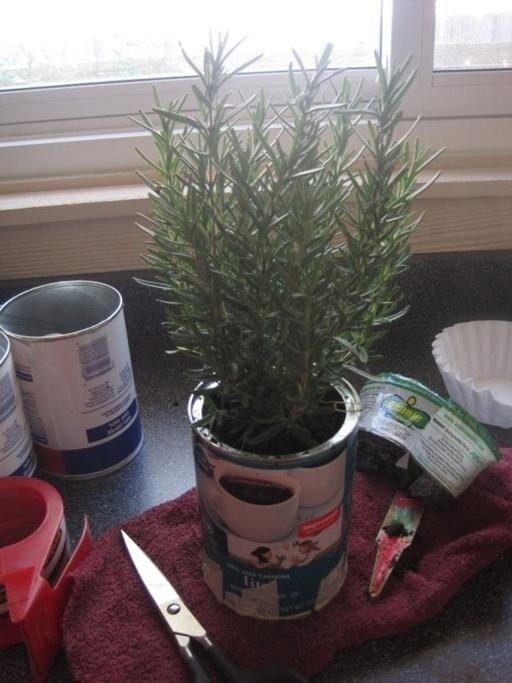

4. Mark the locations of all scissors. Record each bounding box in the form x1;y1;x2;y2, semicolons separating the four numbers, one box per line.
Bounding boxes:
119;530;243;683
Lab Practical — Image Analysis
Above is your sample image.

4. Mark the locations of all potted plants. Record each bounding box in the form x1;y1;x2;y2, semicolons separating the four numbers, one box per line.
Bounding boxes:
119;0;445;625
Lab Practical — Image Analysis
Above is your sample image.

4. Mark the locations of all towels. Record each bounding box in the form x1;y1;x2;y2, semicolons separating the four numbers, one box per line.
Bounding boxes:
61;441;511;681
